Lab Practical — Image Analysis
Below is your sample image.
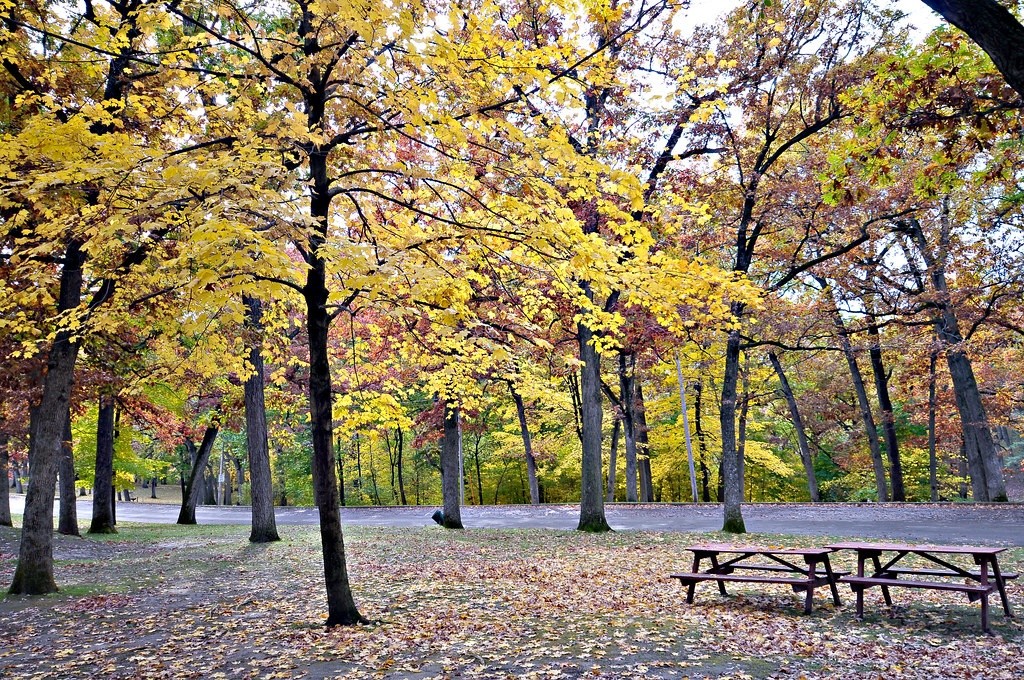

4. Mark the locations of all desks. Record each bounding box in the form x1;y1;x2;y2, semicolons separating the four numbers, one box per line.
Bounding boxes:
685;543;843;614
824;541;1014;632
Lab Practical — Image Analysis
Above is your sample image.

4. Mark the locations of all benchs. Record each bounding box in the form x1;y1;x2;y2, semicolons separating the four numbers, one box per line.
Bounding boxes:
875;568;1020;585
726;563;852;580
670;571;815;593
838;575;992;604
